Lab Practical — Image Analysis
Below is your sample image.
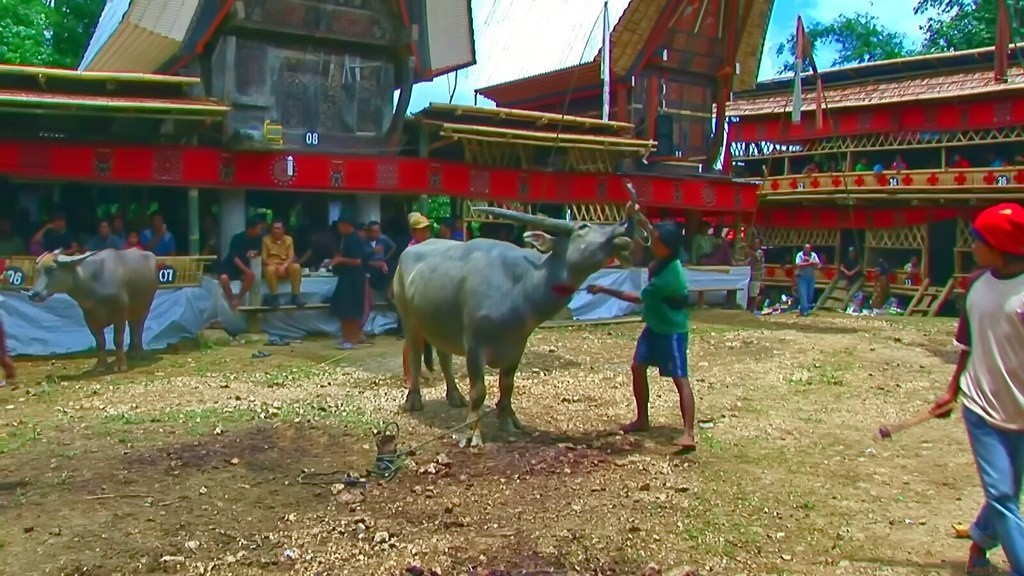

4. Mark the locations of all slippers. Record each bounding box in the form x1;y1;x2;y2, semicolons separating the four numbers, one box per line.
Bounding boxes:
965;556;992;576
249;349;272;360
263;335;290;347
335;341;358;350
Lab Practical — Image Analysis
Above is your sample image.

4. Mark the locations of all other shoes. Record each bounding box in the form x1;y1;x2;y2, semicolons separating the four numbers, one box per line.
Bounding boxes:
355;339;375;347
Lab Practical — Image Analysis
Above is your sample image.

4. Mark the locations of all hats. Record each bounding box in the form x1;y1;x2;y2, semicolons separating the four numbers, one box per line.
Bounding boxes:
408;215;431;231
973;202;1024;257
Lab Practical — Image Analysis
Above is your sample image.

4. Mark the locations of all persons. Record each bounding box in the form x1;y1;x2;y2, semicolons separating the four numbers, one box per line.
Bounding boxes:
0;138;1023;328
584;201;710;447
876;199;1024;576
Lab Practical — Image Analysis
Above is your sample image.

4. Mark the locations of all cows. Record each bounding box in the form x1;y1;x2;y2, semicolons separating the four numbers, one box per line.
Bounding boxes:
391;205;634;434
27;247;159;372
